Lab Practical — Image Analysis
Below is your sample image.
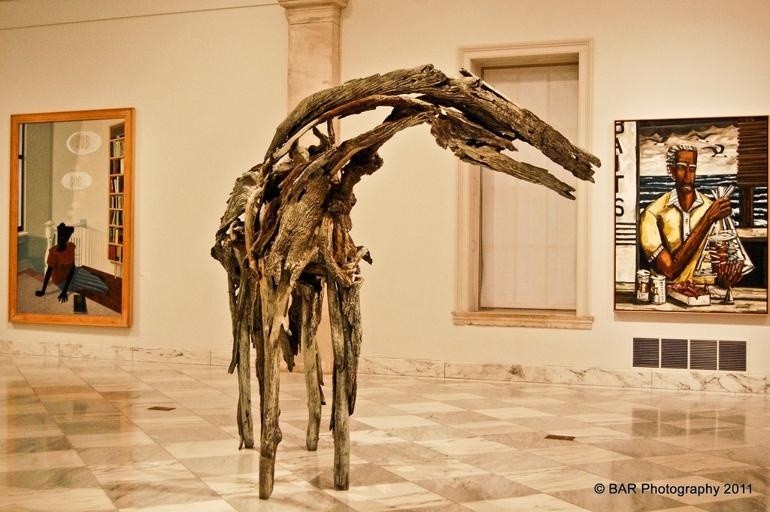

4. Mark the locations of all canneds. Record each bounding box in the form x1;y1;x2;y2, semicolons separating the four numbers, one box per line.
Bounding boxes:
650;274;666;305
636;269;651;302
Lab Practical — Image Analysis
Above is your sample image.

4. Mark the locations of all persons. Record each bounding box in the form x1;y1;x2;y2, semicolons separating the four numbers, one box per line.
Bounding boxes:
640;144;733;284
34;222;109;303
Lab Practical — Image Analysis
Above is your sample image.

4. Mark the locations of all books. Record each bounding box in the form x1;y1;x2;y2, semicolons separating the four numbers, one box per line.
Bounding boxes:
108;135;124;262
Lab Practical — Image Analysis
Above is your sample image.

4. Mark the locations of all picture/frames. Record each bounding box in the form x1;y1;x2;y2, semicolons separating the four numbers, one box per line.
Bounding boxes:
8;105;136;330
